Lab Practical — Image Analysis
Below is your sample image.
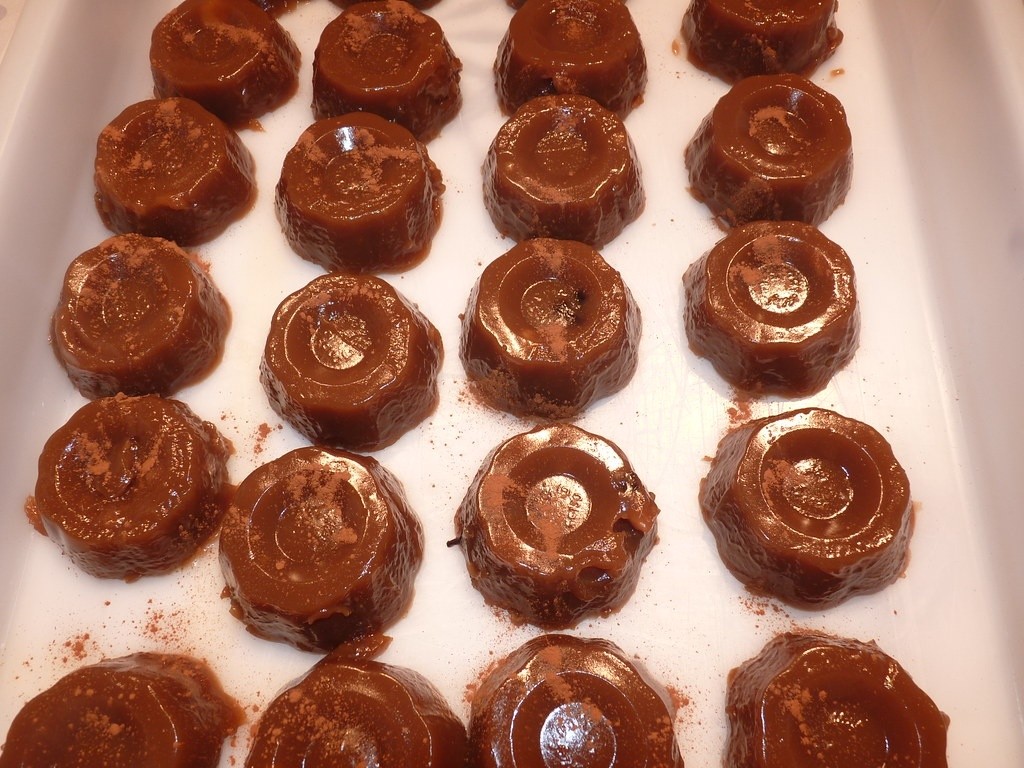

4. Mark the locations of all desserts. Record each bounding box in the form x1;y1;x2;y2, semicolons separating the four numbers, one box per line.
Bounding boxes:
0;0;949;768
